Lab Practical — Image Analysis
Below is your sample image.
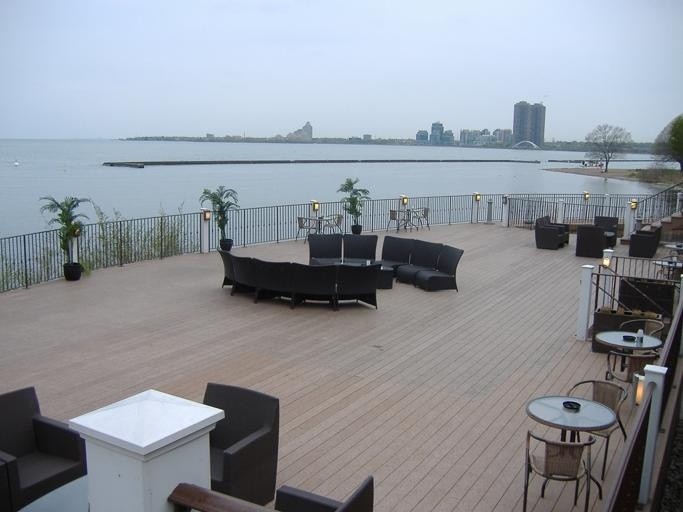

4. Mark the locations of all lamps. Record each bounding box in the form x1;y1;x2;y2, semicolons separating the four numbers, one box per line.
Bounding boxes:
376;267;394;290
476;194;481;201
313;202;319;211
204;211;211;220
632;374;646;407
632;202;637;209
584;193;590;200
603;258;610;268
402;197;408;205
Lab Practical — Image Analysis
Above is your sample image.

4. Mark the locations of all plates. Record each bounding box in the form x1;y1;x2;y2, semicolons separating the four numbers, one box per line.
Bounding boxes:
563;401;580;409
623;336;636;341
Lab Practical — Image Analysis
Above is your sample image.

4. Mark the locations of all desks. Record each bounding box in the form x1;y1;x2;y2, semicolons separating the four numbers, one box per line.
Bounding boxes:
595;332;663;372
526;395;616;500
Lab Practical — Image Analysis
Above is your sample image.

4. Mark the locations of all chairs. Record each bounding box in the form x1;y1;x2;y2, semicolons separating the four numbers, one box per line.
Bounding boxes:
231;253;252;296
202;382;279;506
576;225;607;257
268;476;374;512
629;225;662;257
215;247;232;287
566;380;627;478
619;319;664;341
308;234;343;260
607;351;660;401
397;240;443;288
343;235;378;260
411;207;431;231
417;245;464;293
290;263;336;311
522;431;595;512
337;264;379;310
594;216;619;245
385;210;408;231
535;218;563;250
2;387;88;512
378;234;414;275
252;257;291;306
295;217;320;245
323;214;344;235
543;216;570;247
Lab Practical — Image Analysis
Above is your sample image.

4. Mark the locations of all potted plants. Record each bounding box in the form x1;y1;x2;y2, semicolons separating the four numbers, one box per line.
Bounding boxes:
337;177;372;234
199;186;240;252
40;195;89;281
